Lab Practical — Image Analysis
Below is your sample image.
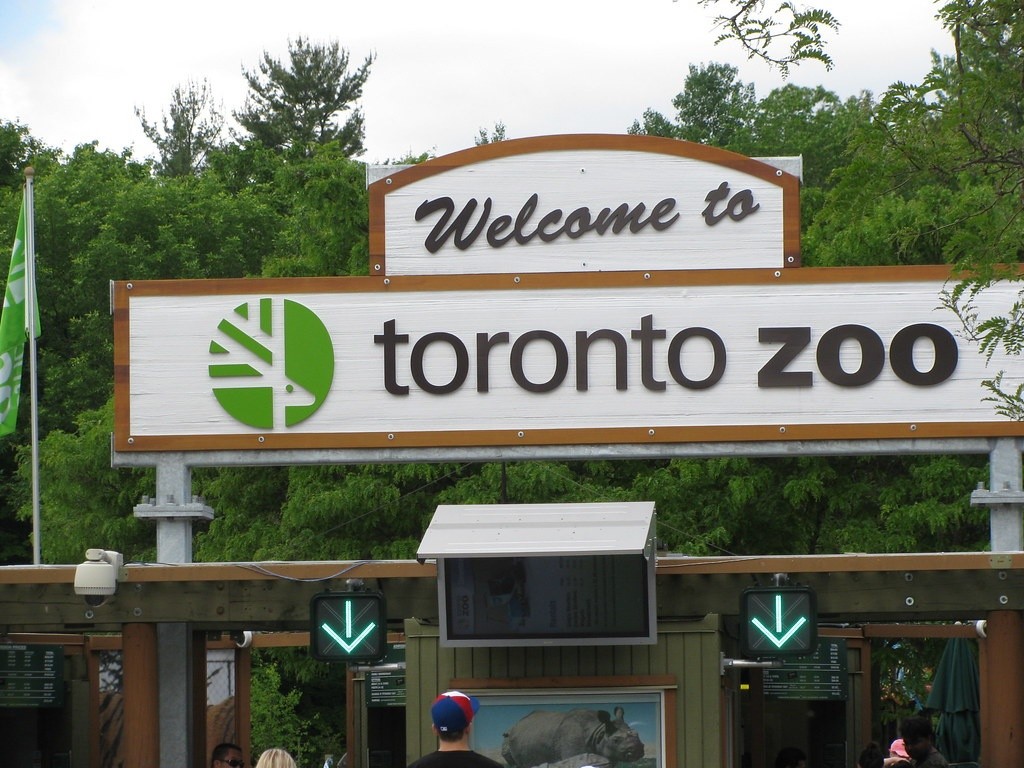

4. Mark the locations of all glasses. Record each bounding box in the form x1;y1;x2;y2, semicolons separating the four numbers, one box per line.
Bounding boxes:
224;759;245;768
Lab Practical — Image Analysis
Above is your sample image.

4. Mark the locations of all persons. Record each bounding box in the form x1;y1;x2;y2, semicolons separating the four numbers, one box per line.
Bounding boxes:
211;743;243;768
255;749;297;768
406;691;505;767
775;748;807;768
858;742;884;768
899;716;947;768
885;739;912;768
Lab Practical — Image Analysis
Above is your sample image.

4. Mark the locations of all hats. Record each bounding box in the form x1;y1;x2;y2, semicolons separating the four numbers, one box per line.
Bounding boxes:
432;690;480;732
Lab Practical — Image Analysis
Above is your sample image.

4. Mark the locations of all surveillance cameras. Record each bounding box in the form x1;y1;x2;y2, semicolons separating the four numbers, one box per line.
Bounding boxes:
73;560;116;608
